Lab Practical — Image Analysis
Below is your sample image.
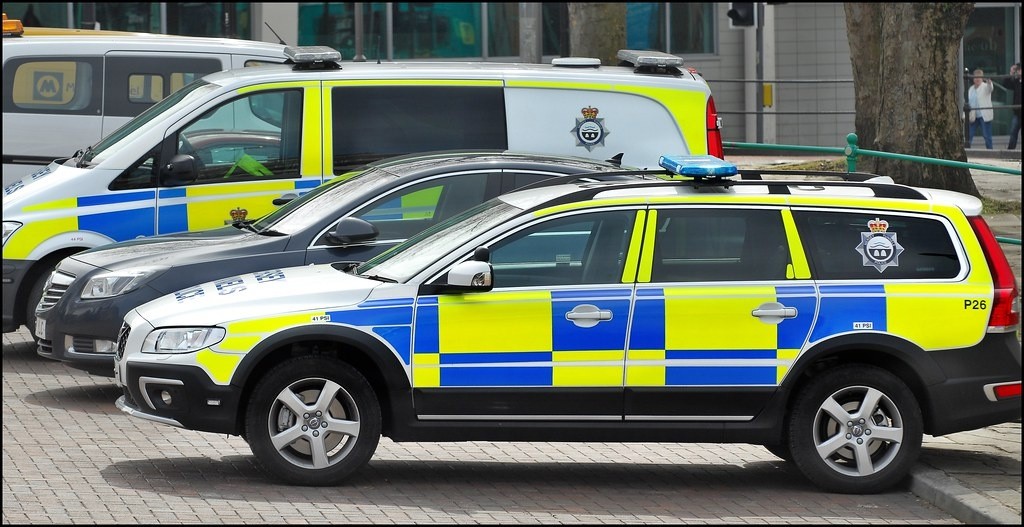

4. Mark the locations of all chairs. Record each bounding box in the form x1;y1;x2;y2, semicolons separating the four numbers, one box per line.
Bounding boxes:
575;237;620;285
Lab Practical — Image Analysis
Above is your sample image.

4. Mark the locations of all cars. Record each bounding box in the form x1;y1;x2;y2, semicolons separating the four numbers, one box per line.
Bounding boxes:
32;153;625;377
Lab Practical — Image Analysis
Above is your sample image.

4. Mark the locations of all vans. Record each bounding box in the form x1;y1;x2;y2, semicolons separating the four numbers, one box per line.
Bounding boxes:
1;43;728;330
1;6;339;197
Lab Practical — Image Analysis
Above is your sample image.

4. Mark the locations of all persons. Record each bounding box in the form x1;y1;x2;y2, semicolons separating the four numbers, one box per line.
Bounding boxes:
1002;64;1022;150
964;69;994;149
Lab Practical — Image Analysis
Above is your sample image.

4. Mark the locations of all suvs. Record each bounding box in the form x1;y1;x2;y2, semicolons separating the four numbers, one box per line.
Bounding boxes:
105;153;1021;494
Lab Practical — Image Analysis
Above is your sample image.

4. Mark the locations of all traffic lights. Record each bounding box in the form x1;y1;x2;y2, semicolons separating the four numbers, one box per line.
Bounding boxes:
725;1;755;28
